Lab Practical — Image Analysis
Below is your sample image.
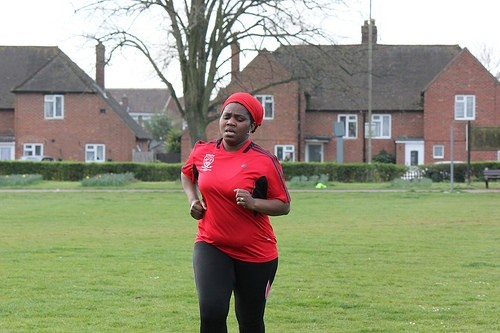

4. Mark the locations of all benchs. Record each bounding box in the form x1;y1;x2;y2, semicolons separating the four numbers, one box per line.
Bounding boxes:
483;170;500;189
